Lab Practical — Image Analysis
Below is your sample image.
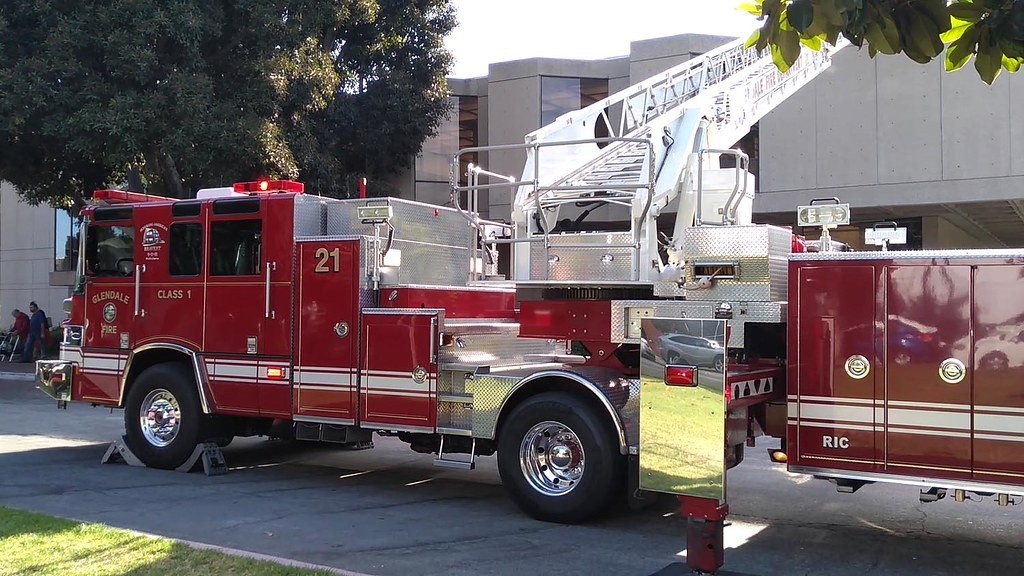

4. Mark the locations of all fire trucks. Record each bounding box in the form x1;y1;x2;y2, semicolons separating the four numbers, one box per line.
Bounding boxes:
30;21;1024;531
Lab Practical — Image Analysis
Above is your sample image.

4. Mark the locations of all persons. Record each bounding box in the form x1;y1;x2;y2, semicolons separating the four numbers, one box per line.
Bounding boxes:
32;318;53;360
18;301;47;363
9;309;30;358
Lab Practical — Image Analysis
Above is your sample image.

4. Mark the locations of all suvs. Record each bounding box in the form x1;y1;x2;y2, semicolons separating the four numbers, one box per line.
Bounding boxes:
654;331;724;376
